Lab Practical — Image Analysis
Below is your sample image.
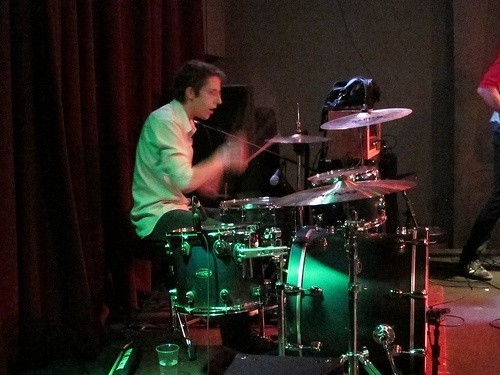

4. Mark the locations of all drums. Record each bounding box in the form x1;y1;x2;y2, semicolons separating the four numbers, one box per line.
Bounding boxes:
282;224;429;375
220;197;293;281
166;224;263;317
307;165;388;232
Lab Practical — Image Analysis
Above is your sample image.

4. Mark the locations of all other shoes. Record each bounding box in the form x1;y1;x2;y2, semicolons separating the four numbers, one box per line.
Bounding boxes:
222;335;276;355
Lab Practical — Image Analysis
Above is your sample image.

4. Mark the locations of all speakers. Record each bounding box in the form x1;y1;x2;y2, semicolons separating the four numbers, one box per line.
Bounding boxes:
222;352;344;375
202;83;256;187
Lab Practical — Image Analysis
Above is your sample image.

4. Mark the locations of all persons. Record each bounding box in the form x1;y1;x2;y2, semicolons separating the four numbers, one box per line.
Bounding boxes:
129;59;278;342
455;58;500;280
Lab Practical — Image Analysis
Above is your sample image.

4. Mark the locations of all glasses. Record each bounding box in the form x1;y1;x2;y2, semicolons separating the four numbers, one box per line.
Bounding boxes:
202;88;222;98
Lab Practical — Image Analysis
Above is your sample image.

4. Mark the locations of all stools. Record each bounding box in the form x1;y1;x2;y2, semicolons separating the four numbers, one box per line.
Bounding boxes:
128;239;207;347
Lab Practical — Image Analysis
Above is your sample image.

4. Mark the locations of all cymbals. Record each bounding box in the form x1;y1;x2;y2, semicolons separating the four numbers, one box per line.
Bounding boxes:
267;135;331;144
321;108;413;132
276;179;417;207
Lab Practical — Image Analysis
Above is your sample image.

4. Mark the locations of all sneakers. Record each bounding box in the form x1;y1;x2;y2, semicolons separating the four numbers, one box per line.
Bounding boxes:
454;260;492;280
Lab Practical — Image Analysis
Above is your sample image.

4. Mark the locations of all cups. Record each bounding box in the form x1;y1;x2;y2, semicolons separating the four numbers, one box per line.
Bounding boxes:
155;344;180;375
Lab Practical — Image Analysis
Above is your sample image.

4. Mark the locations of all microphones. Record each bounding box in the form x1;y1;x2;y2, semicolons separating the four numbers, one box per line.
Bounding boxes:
192;195;207;221
269;158;287;186
